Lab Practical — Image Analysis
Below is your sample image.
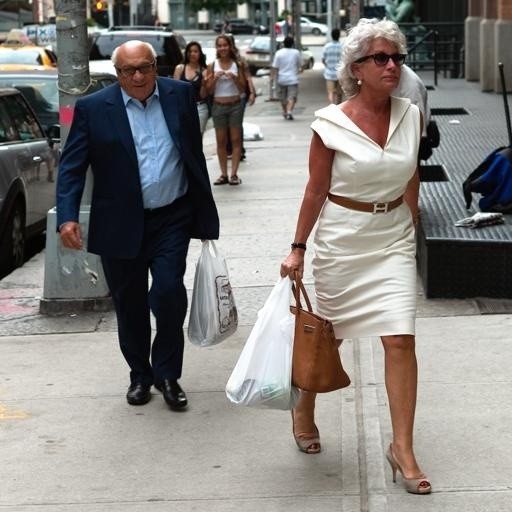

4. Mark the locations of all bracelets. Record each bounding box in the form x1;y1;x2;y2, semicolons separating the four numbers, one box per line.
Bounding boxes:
291;242;306;251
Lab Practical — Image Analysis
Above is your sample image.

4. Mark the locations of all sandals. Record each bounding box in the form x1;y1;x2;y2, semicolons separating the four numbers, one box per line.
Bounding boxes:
213;175;228;185
230;175;239;184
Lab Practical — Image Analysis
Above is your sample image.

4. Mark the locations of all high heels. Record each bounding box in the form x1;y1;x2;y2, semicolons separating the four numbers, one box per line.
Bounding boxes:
291;406;321;454
385;442;432;494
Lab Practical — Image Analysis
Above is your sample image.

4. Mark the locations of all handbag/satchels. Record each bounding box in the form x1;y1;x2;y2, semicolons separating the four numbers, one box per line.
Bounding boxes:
290;270;351;394
463;146;512;214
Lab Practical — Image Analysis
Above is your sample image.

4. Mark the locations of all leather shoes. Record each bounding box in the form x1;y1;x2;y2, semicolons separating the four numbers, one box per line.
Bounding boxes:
126;382;151;405
154;378;187;407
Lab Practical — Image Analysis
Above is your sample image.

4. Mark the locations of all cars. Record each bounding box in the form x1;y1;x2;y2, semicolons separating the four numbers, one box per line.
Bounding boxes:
277;16;328;37
245;35;314;75
212;19;268;36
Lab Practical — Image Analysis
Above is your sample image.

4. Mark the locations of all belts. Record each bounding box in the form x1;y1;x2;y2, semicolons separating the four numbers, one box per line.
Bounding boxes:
196;101;206;105
327;192;403;214
213;99;241;106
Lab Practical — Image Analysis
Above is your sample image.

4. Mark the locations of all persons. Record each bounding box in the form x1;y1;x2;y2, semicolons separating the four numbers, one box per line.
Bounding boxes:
173;42;209;137
206;35;246;186
215;33;255;161
321;29;345;106
55;40;220;408
280;17;431;493
272;36;305;119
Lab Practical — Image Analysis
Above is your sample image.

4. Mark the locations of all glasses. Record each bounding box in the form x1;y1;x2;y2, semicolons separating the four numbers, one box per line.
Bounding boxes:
113;60;155;76
354;52;406;66
